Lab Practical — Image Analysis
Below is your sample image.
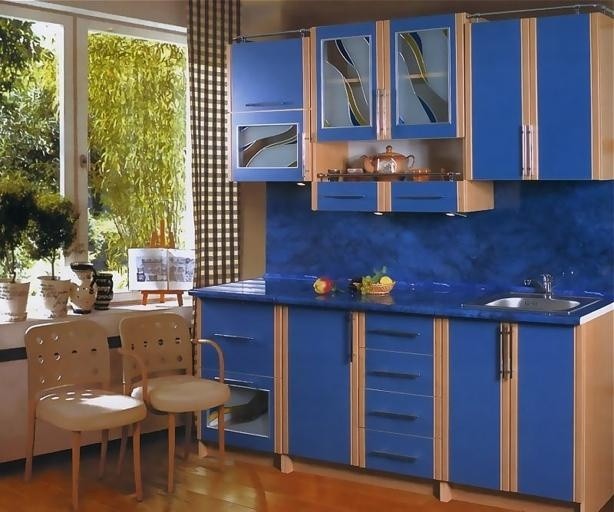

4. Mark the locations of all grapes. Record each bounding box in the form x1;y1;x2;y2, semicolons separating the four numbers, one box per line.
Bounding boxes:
361;276;373;295
349;277;361;285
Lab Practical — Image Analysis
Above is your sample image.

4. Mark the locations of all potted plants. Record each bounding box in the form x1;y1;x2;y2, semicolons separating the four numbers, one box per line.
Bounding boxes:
1;172;80;323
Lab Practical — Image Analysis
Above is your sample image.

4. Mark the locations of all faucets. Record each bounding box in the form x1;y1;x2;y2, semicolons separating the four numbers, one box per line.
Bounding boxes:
522;271;552;296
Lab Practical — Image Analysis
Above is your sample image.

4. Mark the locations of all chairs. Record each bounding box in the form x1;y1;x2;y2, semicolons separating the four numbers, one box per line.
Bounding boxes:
113;317;230;489
23;326;145;506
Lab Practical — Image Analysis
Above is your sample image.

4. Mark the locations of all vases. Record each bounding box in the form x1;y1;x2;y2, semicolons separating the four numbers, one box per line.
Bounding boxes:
68;262;113;314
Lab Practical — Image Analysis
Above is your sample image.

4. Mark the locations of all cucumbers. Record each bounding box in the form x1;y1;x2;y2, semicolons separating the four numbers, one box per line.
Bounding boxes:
371;273;383;284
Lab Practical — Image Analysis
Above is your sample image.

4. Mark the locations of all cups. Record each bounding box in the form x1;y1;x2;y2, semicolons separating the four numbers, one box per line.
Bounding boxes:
69;261;113;315
328;169;340;182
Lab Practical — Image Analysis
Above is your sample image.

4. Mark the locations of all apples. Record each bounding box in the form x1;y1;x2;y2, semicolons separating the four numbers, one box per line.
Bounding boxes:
313;278;332;295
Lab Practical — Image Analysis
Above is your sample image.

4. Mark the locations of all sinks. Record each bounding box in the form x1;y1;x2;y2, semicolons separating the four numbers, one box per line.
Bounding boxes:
459;290;602;314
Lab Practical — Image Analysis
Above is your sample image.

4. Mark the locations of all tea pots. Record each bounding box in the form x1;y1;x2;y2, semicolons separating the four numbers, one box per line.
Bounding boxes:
360;145;415;181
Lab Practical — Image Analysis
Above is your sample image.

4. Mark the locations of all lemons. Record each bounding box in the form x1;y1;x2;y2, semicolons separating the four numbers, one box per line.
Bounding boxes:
380;276;393;285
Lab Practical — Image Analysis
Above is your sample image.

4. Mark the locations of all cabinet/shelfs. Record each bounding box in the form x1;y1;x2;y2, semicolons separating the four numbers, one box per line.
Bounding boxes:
464;12;614;181
358;312;442;482
230;37;311;182
442;310;614;512
194;299;358;467
310;13;494;213
